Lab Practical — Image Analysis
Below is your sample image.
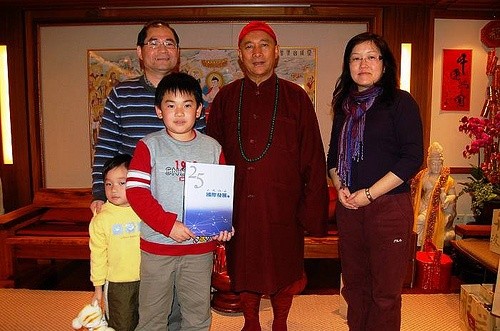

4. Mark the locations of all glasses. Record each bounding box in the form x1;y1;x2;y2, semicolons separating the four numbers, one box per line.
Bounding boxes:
141;38;179;50
348;53;385;64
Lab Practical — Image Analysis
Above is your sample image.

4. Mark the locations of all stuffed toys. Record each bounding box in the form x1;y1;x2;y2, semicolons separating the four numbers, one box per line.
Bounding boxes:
71;299;115;331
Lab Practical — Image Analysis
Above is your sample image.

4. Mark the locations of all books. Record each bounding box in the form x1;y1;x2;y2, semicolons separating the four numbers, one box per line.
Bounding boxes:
182;162;235;236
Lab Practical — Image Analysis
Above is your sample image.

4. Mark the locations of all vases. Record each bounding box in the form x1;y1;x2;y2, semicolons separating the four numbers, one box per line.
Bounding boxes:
472;196;500;223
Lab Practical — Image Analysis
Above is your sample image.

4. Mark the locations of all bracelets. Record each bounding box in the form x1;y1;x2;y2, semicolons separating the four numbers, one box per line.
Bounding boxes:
365;188;375;202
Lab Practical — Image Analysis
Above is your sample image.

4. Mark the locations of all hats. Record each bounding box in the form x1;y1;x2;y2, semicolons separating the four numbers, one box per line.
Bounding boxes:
238;22;276;48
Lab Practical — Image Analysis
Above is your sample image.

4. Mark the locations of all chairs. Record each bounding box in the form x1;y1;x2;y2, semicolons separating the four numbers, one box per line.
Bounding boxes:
450;225;500;275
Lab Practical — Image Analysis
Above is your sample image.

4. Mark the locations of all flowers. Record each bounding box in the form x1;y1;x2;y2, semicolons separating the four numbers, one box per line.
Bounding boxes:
445;116;500;217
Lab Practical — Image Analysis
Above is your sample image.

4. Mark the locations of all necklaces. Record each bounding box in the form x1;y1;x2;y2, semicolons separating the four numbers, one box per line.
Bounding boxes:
238;76;279;162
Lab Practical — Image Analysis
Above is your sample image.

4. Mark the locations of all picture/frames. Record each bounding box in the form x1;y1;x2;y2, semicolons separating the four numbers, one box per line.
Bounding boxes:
86;46;319;168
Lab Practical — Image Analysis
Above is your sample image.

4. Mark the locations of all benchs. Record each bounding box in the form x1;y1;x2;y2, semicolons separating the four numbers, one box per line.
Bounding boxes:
0;188;418;289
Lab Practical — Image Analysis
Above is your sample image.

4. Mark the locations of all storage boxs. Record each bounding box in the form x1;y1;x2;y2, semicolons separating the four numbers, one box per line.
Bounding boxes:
460;284;500;331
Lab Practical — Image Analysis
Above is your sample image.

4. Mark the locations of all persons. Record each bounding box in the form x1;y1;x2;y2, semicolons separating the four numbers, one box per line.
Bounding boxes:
89;154;143;331
416;142;456;252
90;22;207;215
126;72;235;331
206;22;329;331
326;32;423;331
